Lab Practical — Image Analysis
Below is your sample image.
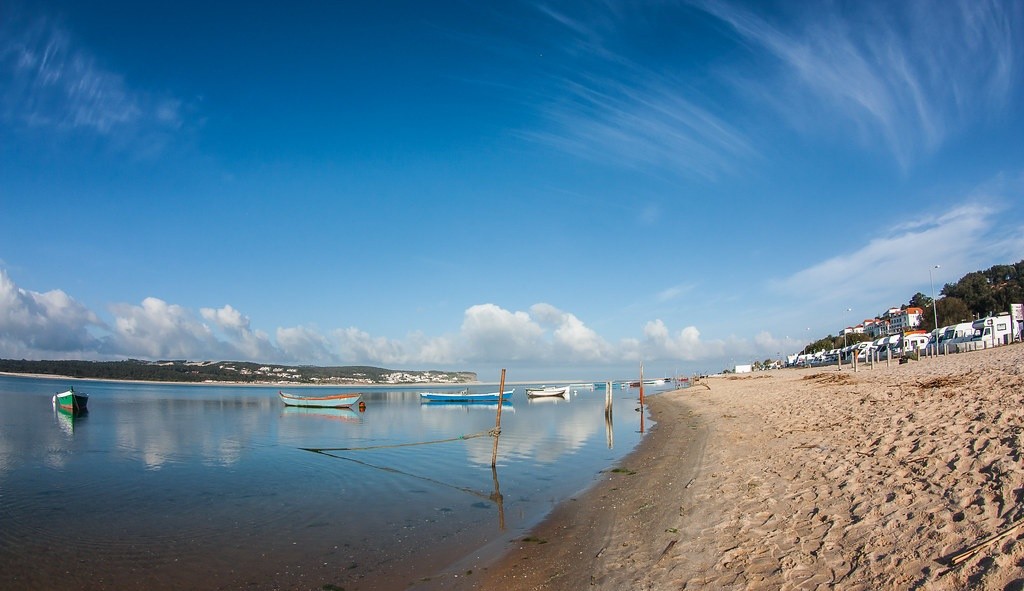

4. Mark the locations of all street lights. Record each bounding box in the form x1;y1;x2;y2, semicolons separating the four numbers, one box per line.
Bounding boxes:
928;265;940;357
843;307;854;361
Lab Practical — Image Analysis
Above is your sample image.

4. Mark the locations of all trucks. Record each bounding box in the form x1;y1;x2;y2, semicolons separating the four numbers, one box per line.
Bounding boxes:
760;313;1024;368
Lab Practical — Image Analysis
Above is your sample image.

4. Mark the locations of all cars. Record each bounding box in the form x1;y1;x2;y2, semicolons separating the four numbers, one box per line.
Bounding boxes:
57;406;88;436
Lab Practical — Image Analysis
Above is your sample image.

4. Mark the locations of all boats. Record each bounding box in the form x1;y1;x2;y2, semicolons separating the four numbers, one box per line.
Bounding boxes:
529;395;568;404
420;388;517;402
281;405;361;423
422;400;516;414
278;390;366;407
51;384;89;410
594;382;621;391
526;385;572;397
